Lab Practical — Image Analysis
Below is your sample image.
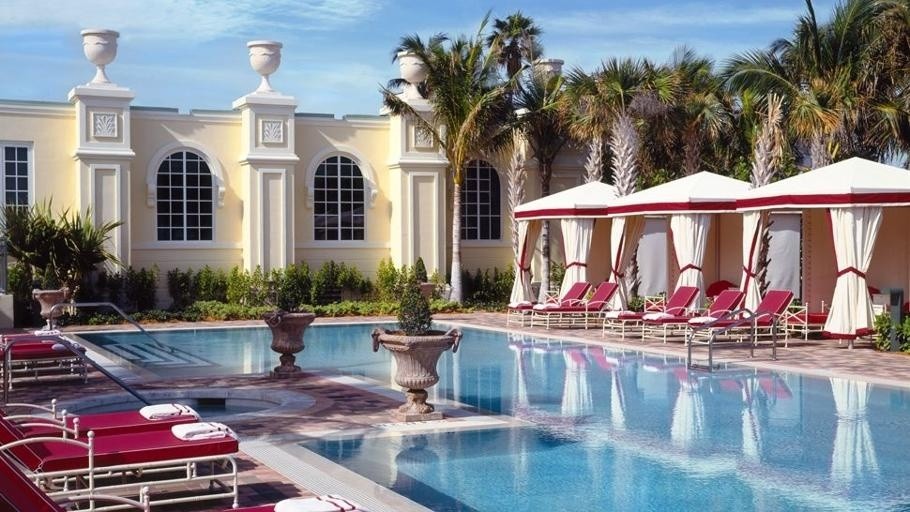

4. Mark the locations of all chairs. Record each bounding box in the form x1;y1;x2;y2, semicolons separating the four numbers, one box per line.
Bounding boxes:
1;330;89;392
507;282;619;330
0;449;358;512
507;333;792;399
0;399;241;512
619;286;794;347
